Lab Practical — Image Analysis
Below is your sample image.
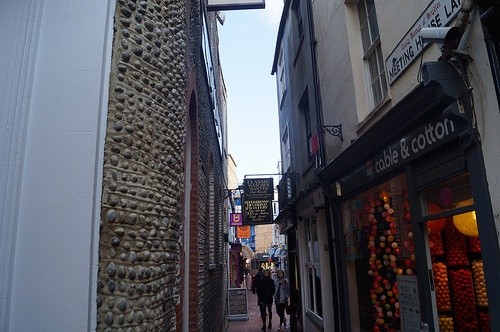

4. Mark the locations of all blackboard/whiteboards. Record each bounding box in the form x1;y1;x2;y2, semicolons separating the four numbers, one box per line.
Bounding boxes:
242;197;274;225
228;288;248;316
245;178;274;199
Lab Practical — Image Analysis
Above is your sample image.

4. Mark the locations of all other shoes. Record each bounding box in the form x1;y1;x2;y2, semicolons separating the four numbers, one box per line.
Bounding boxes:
267;323;272;329
277;327;282;331
261;325;266;332
284;321;288;328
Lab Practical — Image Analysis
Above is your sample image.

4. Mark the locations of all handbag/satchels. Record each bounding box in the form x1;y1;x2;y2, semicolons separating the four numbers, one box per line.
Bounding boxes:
286;305;291;315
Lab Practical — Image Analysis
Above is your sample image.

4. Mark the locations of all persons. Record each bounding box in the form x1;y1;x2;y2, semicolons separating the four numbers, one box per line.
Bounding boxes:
251;266;268;317
256;270;274;331
274;270;289;331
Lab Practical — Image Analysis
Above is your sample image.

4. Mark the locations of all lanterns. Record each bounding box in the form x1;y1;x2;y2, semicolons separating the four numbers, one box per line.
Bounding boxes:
453;202;478;236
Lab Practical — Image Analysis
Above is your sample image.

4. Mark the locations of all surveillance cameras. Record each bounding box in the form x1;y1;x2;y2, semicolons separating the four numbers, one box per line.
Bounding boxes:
419;27;463;43
